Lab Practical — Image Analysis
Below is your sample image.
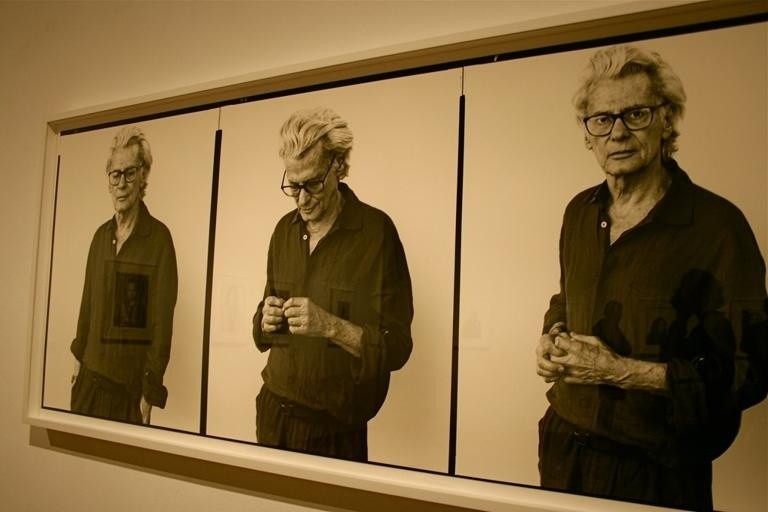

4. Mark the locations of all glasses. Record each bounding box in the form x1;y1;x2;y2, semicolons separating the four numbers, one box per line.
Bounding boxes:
108;165;143;186
281;157;336;198
583;101;668;137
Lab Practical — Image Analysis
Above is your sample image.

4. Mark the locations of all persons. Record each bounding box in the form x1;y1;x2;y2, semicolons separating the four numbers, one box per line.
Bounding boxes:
66;125;178;428
536;46;763;511
251;107;414;464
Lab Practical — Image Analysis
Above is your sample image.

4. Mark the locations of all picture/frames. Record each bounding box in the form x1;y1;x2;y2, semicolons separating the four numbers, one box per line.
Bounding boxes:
26;1;768;512
